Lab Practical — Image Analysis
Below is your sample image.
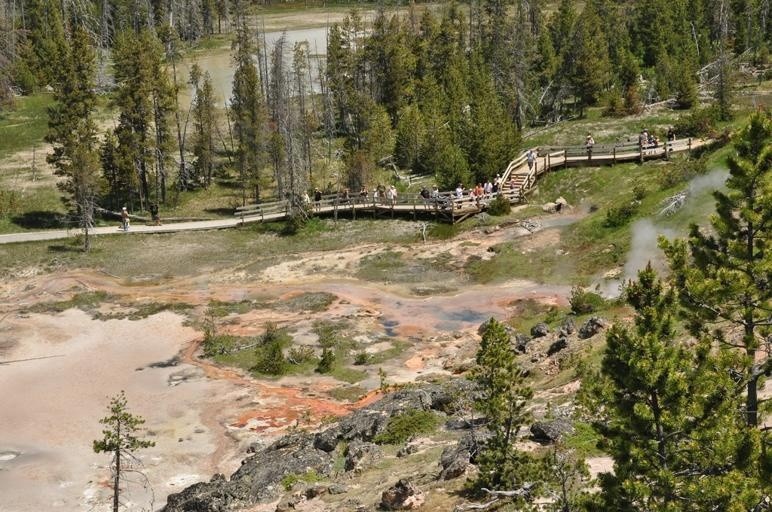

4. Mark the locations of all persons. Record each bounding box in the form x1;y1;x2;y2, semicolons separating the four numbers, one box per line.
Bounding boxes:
666;131;676;152
468;174;503;201
340;186;351;207
526;150;537;171
301;191;309;211
418;182;466;209
314;187;323;212
508;174;518;199
357;183;397;208
149;201;162;226
638;129;660;154
583;132;595;159
121;207;131;231
